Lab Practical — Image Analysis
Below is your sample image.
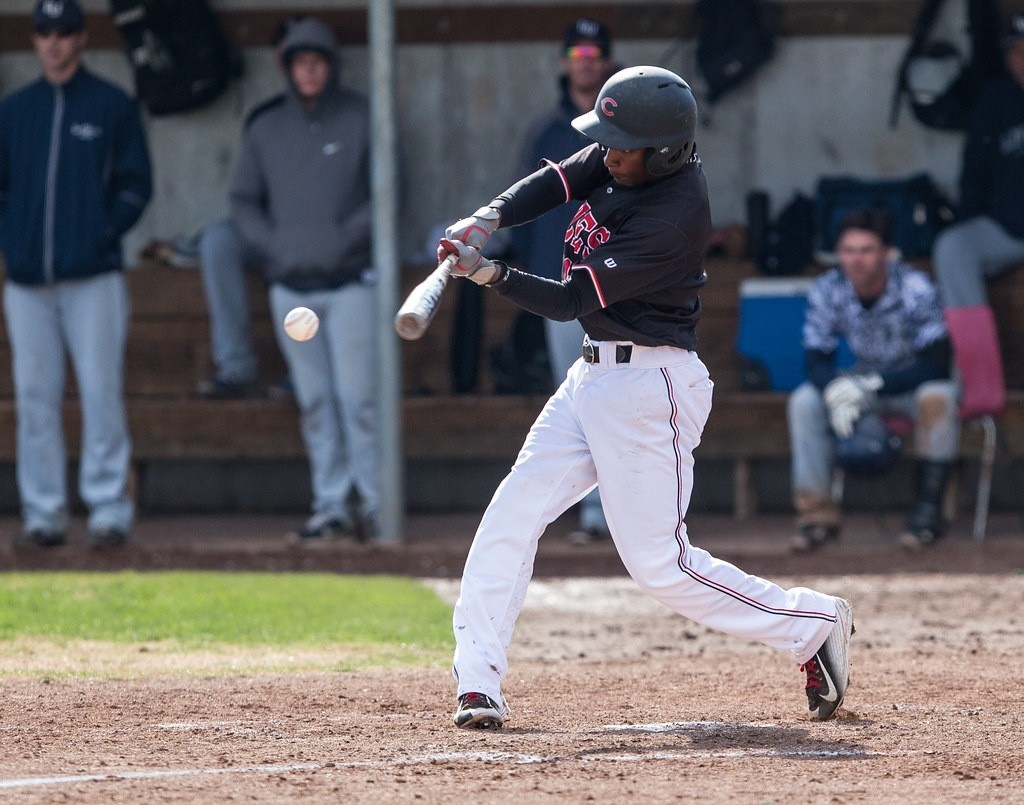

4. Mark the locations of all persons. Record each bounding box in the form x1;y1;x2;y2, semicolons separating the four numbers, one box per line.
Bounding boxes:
197;20;387;542
489;20;627;546
787;211;961;554
434;64;859;736
1;1;155;561
906;13;1024;309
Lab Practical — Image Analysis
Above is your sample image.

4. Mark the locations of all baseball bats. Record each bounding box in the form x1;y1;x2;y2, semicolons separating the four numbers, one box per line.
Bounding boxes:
393;252;459;342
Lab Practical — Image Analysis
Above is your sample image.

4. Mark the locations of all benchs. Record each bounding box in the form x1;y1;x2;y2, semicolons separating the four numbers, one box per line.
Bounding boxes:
0;267;1024;523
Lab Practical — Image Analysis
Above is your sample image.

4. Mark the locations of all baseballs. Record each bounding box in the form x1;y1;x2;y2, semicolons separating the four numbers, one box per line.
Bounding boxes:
283;306;320;342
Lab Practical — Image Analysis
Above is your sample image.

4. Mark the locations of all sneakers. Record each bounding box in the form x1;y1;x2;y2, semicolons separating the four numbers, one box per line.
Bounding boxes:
454;690;504;728
800;597;856;721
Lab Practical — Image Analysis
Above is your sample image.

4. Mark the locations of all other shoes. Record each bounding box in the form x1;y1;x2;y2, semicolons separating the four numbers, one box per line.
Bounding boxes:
902;516;952;550
298;519;339;543
90;525;128;550
23;526;65;548
198;377;250;400
792;523;833;553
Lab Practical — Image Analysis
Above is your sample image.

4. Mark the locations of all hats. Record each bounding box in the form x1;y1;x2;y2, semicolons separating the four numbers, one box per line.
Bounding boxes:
561;18;609;59
32;0;86;32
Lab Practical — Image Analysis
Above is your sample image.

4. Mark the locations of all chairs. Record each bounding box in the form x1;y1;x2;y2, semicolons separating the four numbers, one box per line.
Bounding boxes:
832;309;1005;539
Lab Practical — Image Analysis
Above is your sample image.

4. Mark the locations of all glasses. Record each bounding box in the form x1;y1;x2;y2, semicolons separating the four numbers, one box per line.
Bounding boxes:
35;28;74;38
566;46;602;61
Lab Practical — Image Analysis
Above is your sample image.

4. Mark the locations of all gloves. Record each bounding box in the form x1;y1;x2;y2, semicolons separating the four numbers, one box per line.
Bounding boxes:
825;370;881;442
444;207;499;252
437;238;497;285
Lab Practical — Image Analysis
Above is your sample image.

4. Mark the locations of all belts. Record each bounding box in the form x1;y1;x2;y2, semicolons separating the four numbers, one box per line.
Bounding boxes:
582;343;632;363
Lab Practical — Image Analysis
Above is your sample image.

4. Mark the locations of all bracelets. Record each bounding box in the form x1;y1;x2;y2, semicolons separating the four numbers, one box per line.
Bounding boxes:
485;260;511;288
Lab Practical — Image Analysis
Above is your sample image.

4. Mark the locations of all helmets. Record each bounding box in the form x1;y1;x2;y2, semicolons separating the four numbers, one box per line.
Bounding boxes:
571;66;697;178
